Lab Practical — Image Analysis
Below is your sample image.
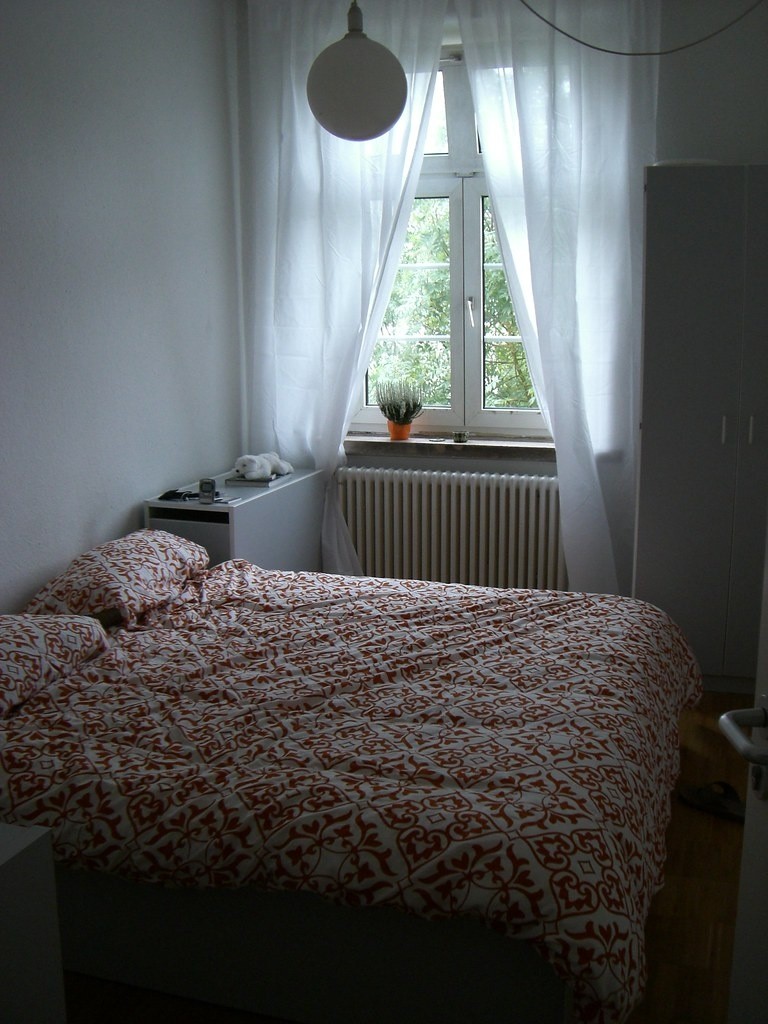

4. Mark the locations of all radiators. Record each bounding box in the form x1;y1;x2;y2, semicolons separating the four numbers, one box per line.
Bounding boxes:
336;464;568;589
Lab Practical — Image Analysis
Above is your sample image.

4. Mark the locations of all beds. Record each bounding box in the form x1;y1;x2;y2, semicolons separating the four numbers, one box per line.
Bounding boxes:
1;525;705;1023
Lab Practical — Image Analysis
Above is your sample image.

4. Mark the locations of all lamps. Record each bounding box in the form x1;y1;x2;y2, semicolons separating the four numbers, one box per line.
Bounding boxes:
306;0;409;143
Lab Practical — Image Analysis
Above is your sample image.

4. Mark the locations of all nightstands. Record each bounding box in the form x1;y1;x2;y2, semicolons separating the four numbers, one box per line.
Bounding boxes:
143;463;324;572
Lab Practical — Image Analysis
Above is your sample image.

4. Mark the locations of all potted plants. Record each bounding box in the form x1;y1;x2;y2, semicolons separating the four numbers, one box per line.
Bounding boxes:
376;380;425;441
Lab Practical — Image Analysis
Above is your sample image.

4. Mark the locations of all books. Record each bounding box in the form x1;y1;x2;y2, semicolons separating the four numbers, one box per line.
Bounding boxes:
225;473;292;488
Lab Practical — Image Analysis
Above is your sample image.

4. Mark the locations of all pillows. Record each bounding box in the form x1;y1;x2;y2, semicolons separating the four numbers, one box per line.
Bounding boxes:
33;529;209;626
0;613;110;713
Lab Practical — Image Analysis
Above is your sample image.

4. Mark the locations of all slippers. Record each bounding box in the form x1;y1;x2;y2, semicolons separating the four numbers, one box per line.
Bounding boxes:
673;773;747;822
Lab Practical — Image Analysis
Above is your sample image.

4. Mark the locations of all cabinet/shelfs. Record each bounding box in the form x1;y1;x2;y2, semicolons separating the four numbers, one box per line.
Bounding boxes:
633;163;768;694
